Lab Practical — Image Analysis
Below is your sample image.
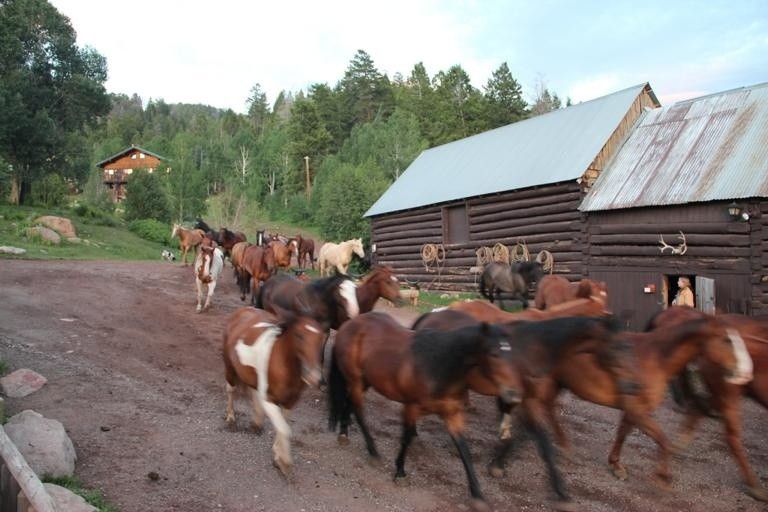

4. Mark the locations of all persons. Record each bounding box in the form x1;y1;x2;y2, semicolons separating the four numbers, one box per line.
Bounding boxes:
672;276;694;309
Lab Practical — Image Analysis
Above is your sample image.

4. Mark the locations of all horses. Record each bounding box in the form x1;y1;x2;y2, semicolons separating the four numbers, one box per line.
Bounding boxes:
221;260;768;512
316;237;365;279
171;219;315;314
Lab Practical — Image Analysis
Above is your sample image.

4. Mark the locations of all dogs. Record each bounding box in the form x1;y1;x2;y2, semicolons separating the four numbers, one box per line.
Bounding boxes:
160;250;176;263
